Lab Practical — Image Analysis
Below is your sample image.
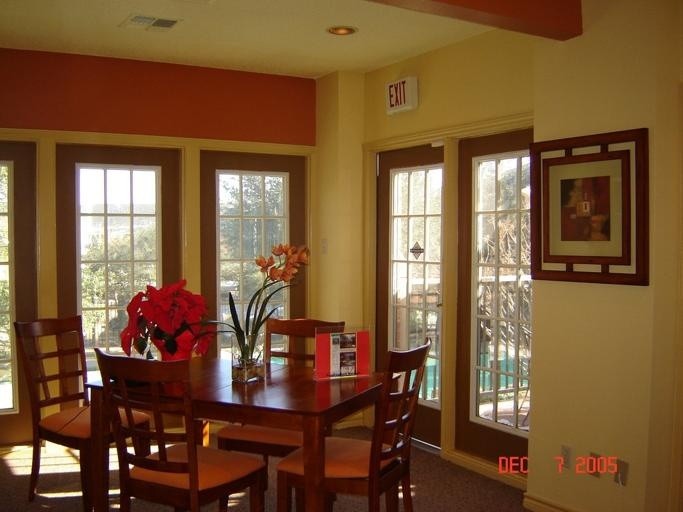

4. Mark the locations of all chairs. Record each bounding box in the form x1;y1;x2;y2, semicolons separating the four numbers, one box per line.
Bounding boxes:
213;316;350;511
92;348;269;511
274;336;434;512
12;316;154;512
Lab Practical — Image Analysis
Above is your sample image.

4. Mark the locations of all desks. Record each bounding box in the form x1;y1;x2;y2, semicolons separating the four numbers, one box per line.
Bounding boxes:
83;357;403;511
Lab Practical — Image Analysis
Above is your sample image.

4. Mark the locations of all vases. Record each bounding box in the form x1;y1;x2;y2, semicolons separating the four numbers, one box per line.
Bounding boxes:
151;331;196;364
227;330;266;386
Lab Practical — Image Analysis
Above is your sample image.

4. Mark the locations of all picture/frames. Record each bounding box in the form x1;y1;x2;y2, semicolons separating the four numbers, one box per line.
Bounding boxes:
529;127;651;287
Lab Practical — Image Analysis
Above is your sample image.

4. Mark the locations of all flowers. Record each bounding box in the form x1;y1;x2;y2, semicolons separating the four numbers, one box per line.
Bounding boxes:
119;279;218;364
191;237;315;366
